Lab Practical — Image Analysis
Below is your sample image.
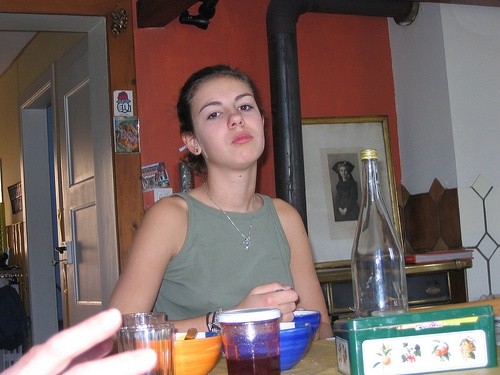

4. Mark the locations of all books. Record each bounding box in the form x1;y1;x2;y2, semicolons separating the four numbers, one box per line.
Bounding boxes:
406;249;474;264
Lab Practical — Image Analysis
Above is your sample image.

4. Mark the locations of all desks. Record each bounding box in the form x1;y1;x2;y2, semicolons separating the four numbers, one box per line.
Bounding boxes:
315;259;473;317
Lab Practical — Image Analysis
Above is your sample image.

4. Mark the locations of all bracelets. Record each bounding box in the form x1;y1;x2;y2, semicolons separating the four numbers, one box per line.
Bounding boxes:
206;308;223;333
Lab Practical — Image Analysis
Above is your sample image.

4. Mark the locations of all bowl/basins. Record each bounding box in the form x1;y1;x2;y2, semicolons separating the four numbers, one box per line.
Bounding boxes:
293;310;320;337
132;332;222;375
222;322;313;370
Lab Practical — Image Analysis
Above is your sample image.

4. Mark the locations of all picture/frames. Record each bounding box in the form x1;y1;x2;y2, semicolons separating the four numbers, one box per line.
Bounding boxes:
113;116;142;154
301;115;404;269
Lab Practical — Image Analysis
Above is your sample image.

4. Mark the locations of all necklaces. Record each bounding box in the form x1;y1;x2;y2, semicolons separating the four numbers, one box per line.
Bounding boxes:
203;184;256;249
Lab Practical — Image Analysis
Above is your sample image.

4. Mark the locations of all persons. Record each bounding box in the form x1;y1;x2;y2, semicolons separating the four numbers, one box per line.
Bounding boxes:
0;308;157;375
108;65;333;340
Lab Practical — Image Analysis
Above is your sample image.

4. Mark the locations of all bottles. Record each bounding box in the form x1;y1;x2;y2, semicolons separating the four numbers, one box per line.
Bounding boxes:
351;148;408;319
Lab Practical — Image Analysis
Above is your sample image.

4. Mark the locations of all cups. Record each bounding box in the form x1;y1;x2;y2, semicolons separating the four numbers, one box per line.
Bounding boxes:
116;323;174;375
218;307;281;375
122;312;166;327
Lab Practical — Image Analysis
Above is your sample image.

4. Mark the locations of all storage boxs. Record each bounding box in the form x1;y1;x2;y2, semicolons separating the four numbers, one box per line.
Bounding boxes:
333;305;497;375
